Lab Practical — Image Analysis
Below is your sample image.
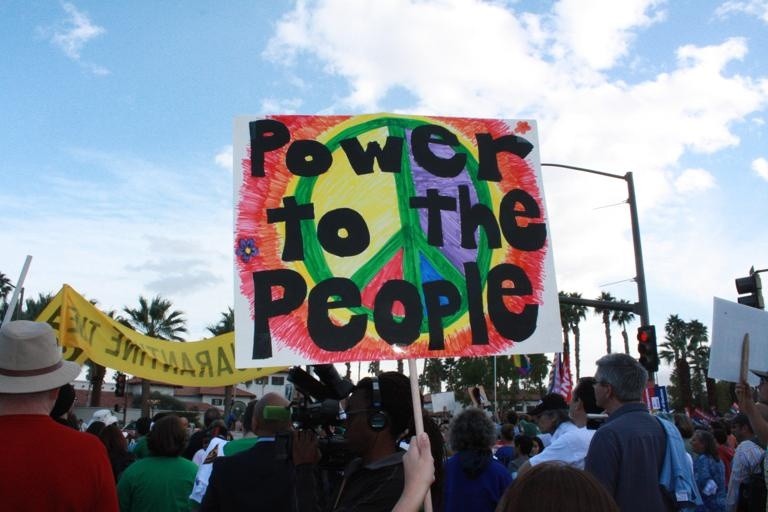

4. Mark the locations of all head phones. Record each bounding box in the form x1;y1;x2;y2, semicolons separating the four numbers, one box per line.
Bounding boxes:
367;376;387;433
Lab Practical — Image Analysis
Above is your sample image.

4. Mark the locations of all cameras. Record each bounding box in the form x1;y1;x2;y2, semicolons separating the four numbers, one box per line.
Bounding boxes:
586;413;609;430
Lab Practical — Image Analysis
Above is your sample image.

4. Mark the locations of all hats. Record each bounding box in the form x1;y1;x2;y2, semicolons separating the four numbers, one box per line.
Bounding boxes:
526;393;564;416
750;369;768;378
87;409;119;430
0;320;81;394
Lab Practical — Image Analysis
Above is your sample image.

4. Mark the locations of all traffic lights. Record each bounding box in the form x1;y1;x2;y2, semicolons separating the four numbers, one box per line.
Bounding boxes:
734;266;764;311
636;325;658;372
114;374;126;397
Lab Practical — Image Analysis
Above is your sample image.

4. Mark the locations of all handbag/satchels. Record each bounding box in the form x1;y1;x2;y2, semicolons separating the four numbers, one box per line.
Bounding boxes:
736;472;767;511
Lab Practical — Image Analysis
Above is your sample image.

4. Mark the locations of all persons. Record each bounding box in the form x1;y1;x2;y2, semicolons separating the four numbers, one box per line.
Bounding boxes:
0;320;119;509
53;354;768;511
